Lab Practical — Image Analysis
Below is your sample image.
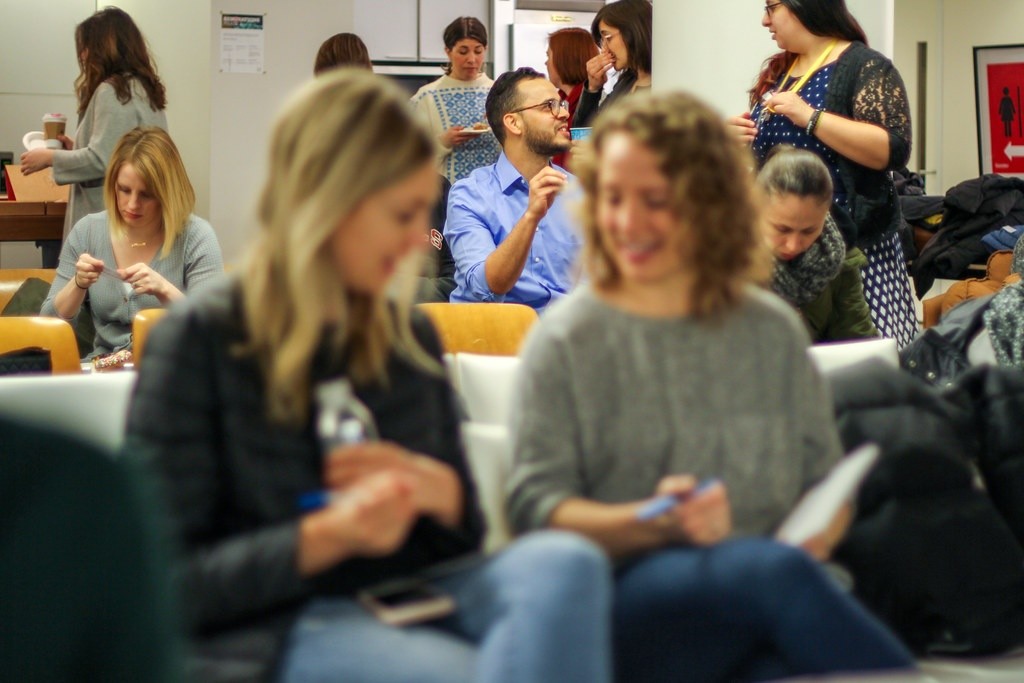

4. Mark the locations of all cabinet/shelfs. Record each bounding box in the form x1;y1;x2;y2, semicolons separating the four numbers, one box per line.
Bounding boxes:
351;0;488;76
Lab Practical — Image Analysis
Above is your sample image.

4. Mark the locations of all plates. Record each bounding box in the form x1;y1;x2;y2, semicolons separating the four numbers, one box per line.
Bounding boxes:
459;126;491;135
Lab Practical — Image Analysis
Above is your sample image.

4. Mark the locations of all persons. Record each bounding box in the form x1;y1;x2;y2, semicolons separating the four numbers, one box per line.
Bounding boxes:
412;173;458;303
408;15;502;186
313;31;374;80
571;0;653;128
39;124;225;362
821;237;1023;659
438;67;592;318
493;85;925;682
723;0;922;352
753;139;882;345
543;26;607;176
16;2;169;245
120;69;614;683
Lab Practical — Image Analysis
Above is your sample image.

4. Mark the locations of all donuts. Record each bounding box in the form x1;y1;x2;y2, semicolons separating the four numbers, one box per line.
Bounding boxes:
92;350;132;371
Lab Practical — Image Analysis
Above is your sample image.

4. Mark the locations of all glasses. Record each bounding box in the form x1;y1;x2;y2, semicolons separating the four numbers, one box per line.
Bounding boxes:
599;32;621;50
500;100;569;126
764;1;783;18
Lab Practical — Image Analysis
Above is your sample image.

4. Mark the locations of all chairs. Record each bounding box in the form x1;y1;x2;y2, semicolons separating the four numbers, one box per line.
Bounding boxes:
0;267;901;683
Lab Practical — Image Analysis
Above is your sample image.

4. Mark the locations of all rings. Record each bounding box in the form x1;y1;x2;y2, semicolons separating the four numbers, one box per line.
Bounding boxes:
134;281;141;287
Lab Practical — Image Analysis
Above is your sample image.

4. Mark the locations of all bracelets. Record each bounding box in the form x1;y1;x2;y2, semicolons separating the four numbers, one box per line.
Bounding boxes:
74;273;88;290
804;109;821;136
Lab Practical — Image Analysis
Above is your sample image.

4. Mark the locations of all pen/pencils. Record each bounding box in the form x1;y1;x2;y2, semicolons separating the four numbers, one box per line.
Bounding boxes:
298;489;331;509
549;160;553;167
637;477;714;520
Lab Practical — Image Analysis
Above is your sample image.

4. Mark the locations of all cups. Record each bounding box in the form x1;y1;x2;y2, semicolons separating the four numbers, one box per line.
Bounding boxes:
42;111;67;150
570;127;593;140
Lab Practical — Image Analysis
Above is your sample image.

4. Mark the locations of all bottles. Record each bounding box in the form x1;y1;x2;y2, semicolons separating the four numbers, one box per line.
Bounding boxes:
314;376;379;450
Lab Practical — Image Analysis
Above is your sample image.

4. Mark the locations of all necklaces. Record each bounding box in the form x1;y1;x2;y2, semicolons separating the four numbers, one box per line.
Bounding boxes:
123;234;152;248
